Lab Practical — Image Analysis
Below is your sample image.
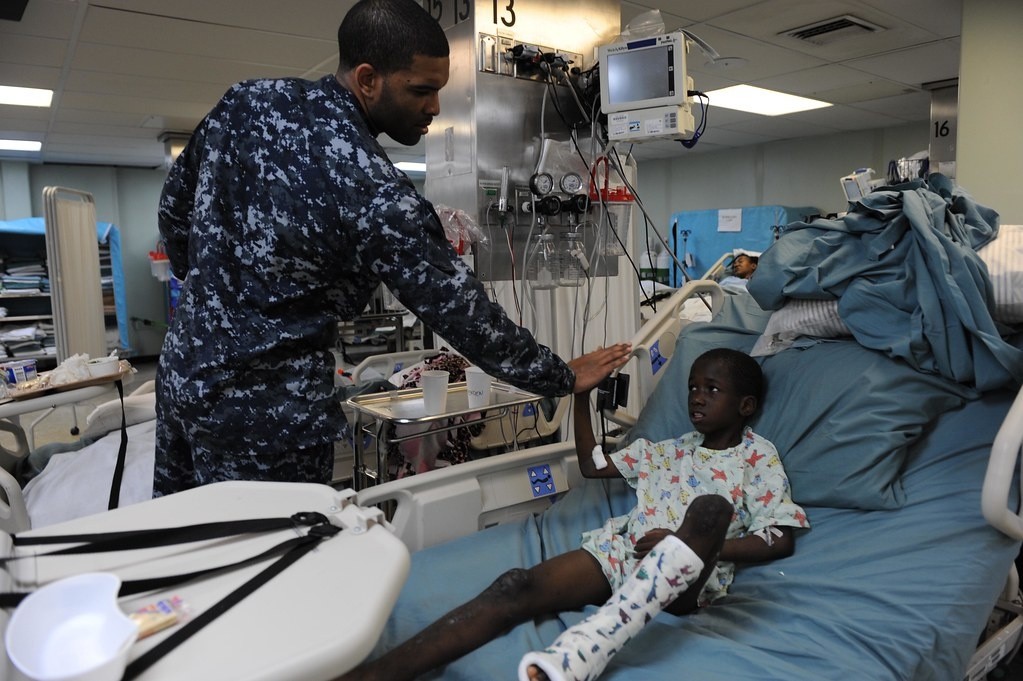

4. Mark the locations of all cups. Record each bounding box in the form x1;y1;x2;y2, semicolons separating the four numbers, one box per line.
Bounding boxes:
420;370;450;414
465;366;490;408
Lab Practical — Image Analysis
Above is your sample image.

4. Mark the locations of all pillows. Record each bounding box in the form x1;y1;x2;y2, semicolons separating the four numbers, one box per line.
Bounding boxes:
615;321;961;513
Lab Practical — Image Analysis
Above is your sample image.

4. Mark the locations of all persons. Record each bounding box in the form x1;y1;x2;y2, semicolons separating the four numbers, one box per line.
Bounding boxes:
153;0;632;498
716;253;759;293
343;346;810;681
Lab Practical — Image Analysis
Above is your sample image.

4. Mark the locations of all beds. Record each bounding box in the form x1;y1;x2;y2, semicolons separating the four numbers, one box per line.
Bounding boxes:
680;248;762;322
354;247;1022;680
0;349;445;533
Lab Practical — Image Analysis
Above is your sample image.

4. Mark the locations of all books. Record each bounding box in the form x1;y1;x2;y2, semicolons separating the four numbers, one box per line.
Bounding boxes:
0;242;115;360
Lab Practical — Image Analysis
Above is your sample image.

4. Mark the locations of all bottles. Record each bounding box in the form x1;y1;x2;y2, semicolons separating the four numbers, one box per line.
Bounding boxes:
557;233;587;287
529;233;559;290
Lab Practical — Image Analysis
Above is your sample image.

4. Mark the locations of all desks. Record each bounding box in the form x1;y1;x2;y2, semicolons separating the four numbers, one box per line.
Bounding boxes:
0;367;135;420
0;481;411;681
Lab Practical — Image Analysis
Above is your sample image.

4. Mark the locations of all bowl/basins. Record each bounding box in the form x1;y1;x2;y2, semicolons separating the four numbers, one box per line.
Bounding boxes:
5;359;37;384
87;356;119;379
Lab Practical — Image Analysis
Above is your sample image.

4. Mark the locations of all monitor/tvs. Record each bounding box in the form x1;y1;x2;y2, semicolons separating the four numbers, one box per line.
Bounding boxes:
840;167;874;201
598;32;688;114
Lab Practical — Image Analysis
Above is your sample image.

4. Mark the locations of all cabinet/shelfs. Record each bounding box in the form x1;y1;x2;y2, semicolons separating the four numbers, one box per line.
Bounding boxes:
0;216;132;361
